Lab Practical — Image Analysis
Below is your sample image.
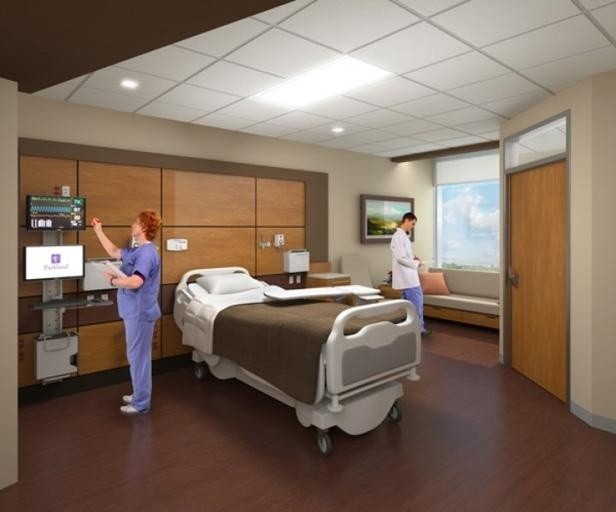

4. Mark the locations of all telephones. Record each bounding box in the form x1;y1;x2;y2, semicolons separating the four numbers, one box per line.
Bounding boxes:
275;234;285;248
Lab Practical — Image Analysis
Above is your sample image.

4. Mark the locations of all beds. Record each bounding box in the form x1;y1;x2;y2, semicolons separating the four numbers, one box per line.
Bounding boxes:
172;265;422;456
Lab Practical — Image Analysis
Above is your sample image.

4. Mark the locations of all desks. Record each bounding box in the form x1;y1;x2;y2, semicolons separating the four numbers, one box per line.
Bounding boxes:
262;285;383;307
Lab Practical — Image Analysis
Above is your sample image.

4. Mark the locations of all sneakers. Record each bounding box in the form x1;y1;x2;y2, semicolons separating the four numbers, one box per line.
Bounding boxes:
422;330;431;337
122;394;133;402
120;405;138;414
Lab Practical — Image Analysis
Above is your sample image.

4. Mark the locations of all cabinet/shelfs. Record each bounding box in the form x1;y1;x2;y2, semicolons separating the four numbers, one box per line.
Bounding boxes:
306;272;353;305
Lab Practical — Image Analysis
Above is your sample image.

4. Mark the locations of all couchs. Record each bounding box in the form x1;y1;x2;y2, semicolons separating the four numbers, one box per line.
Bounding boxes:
419;267;501;329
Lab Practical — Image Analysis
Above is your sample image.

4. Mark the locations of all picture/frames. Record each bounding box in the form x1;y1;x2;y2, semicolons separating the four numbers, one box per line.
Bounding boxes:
360;193;414;245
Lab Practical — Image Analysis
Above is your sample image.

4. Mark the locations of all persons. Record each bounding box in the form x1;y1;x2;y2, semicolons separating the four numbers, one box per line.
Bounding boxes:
390;213;431;337
91;208;164;415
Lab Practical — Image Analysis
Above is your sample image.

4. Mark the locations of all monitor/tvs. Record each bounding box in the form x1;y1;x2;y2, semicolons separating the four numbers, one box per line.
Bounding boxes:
23;244;85;281
26;195;86;230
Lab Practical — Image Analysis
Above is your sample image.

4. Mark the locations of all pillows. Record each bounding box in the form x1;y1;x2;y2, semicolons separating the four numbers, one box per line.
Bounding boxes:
195;273;263;294
420;271;451;295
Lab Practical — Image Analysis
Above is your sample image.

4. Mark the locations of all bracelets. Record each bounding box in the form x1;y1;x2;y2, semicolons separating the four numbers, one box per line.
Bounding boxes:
110;277;117;286
